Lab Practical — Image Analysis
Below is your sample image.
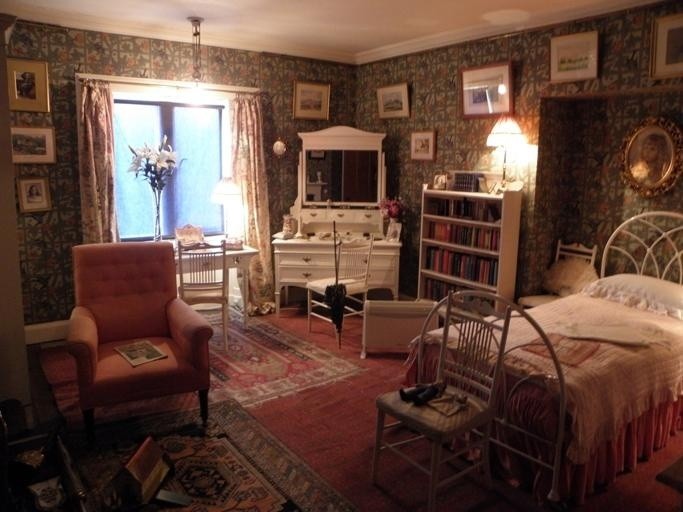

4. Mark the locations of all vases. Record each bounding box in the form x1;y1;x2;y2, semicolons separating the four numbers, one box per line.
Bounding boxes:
154;189;163;243
386;217;398;240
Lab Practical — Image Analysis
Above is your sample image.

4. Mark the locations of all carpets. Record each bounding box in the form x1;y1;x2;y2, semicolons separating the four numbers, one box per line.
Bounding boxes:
43;401;359;512
53;309;357;422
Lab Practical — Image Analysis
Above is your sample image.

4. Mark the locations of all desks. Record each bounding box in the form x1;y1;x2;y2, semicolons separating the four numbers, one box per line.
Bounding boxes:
150;234;260;328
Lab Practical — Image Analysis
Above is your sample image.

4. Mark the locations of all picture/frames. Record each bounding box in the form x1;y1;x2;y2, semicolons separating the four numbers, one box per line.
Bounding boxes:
432;173;446;189
549;30;598;84
17;175;51;213
649;12;683;80
417;169;524;318
376;83;410;119
411;130;437;161
292;79;330;121
456;61;514;118
307;150;326;159
620;114;683;198
9;125;56;164
7;57;49;112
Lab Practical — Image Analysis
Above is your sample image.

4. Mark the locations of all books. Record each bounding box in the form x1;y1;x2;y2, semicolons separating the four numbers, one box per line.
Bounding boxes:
426;246;498;288
428;196;499;224
427;220;499;253
424;276;494;317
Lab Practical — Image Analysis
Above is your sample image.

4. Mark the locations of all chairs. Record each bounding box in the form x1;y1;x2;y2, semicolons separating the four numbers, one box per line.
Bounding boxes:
372;291;512;512
178;240;229;352
518;238;598;311
306;236;374;354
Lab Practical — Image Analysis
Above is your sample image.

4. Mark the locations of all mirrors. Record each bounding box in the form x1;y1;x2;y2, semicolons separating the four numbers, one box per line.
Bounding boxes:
291;126;387;209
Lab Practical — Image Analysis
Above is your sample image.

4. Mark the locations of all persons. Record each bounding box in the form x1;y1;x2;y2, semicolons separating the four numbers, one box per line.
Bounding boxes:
640;134;669;182
126;344;161;360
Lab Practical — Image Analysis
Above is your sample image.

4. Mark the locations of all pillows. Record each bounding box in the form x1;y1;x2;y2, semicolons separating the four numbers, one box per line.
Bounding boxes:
581;273;683;321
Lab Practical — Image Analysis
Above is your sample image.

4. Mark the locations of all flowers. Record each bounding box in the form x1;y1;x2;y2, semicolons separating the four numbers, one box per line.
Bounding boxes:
379;198;408;217
123;134;188;185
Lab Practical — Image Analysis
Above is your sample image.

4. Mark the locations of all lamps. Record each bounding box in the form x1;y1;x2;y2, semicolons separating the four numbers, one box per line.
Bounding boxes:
185;17;209;83
211;177;244;239
486;114;522;183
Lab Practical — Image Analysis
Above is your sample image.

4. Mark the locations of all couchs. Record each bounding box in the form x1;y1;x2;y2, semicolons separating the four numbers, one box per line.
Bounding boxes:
64;242;212;428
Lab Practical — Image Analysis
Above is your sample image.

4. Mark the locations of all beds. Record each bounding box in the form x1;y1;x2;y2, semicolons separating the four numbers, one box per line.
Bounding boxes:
402;212;683;502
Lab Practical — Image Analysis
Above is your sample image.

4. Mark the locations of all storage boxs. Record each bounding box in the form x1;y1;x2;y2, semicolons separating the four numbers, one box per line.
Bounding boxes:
361;301;438;358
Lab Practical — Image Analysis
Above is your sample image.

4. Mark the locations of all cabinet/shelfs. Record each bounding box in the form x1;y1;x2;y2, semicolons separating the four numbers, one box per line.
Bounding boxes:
272;231;401;318
